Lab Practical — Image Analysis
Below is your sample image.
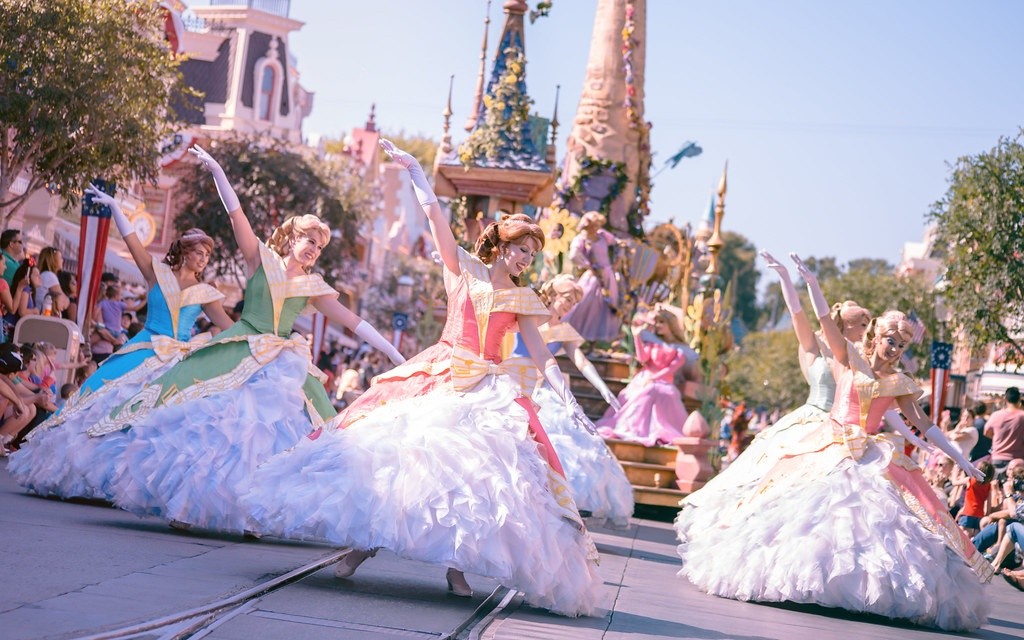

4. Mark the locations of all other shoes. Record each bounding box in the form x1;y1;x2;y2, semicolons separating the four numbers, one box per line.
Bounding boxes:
170;520;191;529
3;448;10;457
6;445;17;453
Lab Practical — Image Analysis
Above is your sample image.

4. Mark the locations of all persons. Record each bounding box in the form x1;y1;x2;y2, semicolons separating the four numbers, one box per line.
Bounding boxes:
0;137;1023;627
65;143;406;542
671;251;987;630
236;137;611;619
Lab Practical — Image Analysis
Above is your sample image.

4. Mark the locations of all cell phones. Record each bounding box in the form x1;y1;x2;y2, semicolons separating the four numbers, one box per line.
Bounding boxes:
27;267;31;278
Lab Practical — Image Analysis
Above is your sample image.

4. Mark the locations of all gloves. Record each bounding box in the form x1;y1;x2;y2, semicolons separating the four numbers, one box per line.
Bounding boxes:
925;425;989;482
188;144;240;213
569;348;622;414
85;183;136;238
354;320;406;367
760;251;802;314
378;138;438;205
543;366;598;436
885;408;936;455
791;254;830;318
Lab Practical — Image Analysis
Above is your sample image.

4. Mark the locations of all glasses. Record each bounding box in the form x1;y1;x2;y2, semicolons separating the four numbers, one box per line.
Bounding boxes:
16;240;23;244
54;248;57;253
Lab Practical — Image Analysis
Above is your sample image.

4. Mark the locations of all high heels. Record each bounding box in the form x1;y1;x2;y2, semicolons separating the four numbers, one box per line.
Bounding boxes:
334;547;379;577
446;568;473;596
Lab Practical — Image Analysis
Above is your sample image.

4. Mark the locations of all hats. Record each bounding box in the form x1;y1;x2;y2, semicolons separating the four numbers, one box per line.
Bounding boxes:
101;272;116;282
0;352;28;373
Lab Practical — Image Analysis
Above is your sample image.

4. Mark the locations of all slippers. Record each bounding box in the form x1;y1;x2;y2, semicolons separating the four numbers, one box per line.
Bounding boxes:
1001;568;1024;592
